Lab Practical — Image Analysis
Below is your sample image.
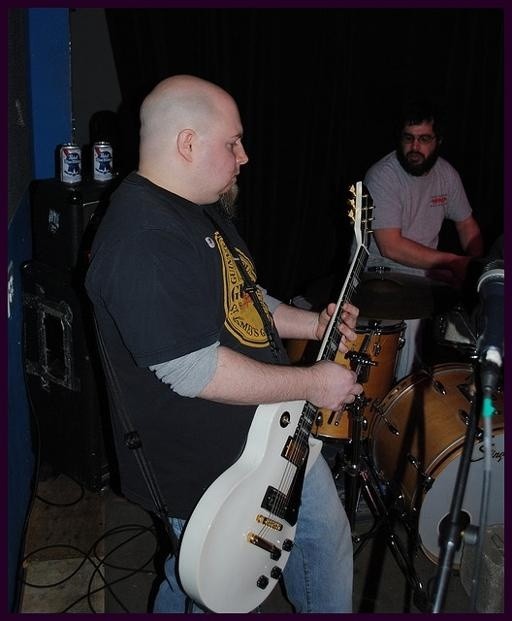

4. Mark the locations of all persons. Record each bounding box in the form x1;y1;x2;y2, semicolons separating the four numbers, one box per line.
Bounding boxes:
85;75;366;614
343;101;502;382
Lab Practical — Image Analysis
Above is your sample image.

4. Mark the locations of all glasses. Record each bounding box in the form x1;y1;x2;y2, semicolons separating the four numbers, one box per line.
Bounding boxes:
404;135;435;144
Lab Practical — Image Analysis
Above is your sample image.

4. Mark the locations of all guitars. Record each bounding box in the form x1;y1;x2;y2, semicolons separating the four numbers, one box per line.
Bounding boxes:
177;182;374;614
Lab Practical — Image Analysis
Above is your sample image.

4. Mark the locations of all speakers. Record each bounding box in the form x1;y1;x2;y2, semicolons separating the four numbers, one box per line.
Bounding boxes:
28;179;120;494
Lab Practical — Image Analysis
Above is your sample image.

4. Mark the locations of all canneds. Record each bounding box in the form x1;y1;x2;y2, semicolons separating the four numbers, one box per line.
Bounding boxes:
58;143;82;183
90;142;114;180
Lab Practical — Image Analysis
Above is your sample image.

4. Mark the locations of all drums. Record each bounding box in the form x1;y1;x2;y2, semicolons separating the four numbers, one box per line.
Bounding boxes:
284;319;406;440
368;362;505;571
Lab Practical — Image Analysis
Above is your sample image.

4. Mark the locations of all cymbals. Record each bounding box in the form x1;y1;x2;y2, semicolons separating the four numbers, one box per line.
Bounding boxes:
293;271;462;320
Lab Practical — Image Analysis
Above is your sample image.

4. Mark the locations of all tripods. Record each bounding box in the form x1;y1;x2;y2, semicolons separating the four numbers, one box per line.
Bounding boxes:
330;384;482;614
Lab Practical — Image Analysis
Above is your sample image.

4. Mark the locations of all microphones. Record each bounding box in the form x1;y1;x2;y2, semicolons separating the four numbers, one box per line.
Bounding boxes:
476;257;505;396
